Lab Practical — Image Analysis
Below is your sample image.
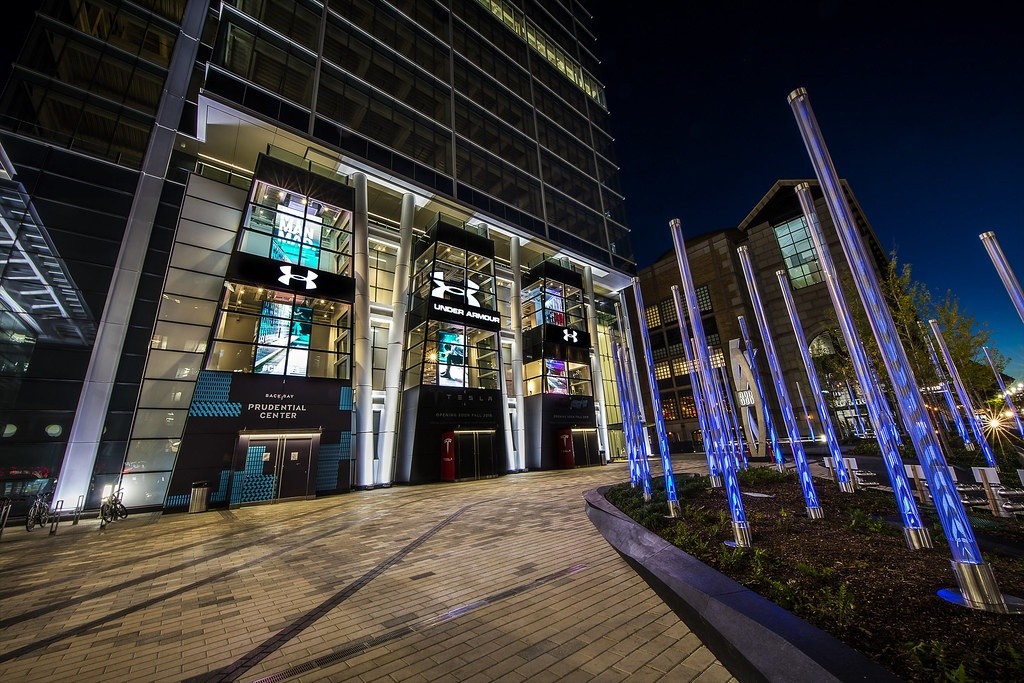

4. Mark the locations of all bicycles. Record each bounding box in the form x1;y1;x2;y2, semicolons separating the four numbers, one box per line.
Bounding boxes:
25;492;50;531
99;487;128;523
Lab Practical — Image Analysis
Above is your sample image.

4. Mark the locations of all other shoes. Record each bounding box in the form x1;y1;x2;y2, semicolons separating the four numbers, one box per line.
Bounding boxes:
440;370;446;374
441;372;450;378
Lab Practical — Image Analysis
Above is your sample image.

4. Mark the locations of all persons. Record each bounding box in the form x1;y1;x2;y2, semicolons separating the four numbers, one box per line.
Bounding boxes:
441;342;468;379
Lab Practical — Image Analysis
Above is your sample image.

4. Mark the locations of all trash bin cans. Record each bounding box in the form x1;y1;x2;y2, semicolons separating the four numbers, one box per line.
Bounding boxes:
188;480;209;513
